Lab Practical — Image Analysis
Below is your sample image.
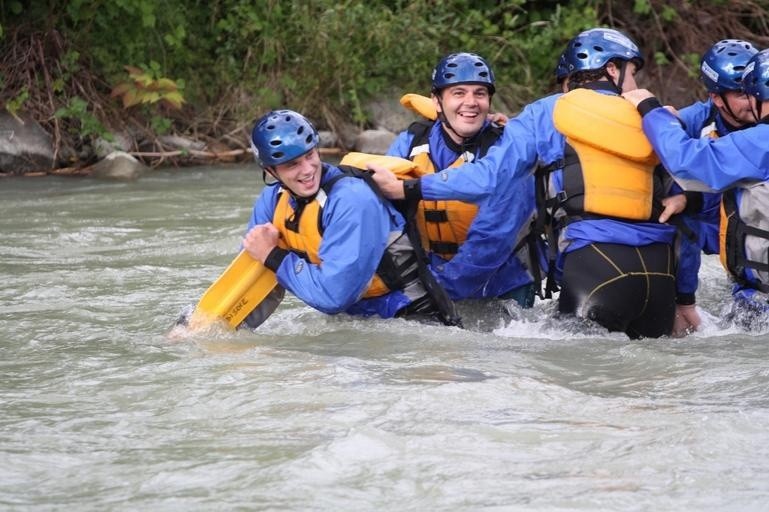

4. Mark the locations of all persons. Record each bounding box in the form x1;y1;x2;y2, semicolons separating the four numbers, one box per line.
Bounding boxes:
165;108;464;336
622;38;768;328
370;27;705;343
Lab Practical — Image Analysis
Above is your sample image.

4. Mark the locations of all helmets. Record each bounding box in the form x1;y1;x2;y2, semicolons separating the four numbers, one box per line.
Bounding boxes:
700;38;769;101
556;28;643;84
431;53;495;94
252;108;319;167
